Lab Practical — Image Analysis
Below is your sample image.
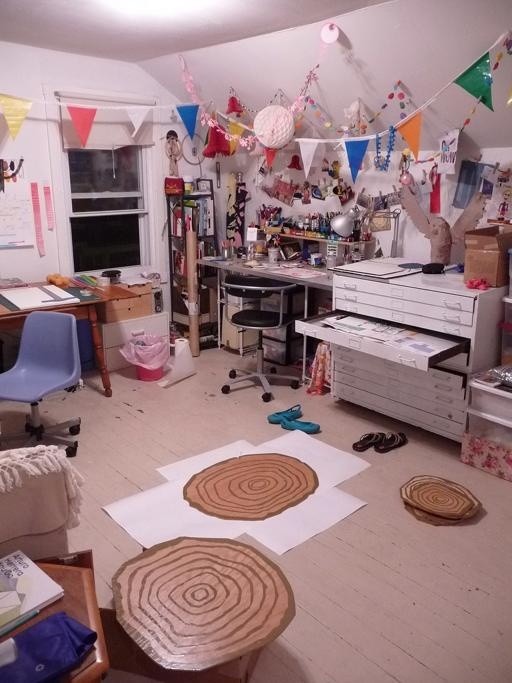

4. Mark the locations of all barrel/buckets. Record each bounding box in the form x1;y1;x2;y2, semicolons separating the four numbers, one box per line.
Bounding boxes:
352;431;385;452
374;432;406;453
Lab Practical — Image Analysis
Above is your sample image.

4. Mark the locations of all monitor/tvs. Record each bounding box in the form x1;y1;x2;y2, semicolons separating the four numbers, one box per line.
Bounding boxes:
0;551;65;639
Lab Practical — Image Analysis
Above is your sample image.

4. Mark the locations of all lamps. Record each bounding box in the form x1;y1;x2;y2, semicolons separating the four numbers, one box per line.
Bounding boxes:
0;311;84;459
222;275;297;402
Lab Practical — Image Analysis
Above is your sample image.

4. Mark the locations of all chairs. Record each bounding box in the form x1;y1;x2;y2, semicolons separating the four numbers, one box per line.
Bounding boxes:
280;415;320;434
267;403;302;424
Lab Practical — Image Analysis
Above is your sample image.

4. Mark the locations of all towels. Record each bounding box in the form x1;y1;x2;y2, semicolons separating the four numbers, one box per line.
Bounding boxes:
129;334;168;382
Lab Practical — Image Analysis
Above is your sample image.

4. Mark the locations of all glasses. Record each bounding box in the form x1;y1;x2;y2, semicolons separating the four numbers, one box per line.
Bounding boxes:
331;205;401;258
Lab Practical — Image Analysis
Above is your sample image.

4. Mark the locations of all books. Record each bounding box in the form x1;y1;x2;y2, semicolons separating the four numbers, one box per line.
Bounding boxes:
467;378;512;424
98;283;155;323
465;407;512;447
459;432;512;481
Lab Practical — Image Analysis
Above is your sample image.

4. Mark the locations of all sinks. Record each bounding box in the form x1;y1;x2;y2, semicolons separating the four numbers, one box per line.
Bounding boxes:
1;444;85;558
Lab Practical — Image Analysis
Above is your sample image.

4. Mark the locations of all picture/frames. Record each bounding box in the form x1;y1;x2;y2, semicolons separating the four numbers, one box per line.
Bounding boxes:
1;279;138;398
196;252;346;393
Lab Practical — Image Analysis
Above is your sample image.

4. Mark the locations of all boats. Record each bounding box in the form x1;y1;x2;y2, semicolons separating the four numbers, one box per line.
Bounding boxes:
151;287;164;314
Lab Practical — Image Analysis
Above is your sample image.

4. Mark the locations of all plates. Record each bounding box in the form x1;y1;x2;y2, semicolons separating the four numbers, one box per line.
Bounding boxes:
99;311;169;374
294;253;507;445
